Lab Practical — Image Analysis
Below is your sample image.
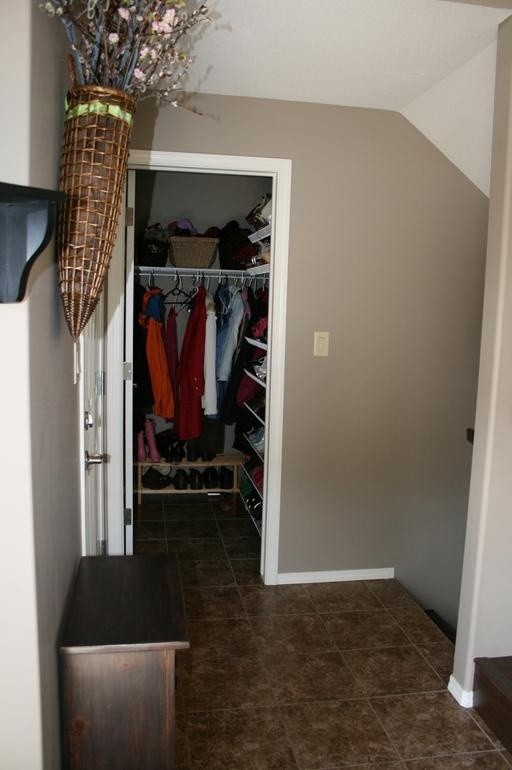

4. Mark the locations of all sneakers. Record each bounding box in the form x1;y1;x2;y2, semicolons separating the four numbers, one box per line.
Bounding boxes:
219;468;232;489
204;467;217;489
172;469;187;489
239;354;267;535
187;469;203;489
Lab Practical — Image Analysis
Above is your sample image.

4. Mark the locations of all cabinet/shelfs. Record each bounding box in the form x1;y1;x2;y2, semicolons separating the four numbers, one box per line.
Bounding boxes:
134;456;243;505
237;224;271;539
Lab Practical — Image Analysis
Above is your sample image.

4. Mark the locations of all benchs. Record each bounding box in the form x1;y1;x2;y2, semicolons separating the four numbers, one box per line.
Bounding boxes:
56;552;191;770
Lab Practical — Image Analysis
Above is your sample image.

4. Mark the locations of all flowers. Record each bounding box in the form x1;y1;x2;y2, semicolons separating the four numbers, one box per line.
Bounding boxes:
38;0;225;124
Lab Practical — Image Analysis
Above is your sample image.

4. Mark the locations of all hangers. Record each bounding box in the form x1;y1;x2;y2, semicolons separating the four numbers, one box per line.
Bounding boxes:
134;268;268;315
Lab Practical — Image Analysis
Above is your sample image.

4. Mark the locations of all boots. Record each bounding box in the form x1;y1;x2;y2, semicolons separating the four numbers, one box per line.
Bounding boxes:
156;428;216;462
137;431;146;461
145;419;160;462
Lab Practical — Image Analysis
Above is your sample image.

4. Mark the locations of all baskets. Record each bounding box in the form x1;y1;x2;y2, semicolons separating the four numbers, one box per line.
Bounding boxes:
170;236;220;269
56;86;137;340
